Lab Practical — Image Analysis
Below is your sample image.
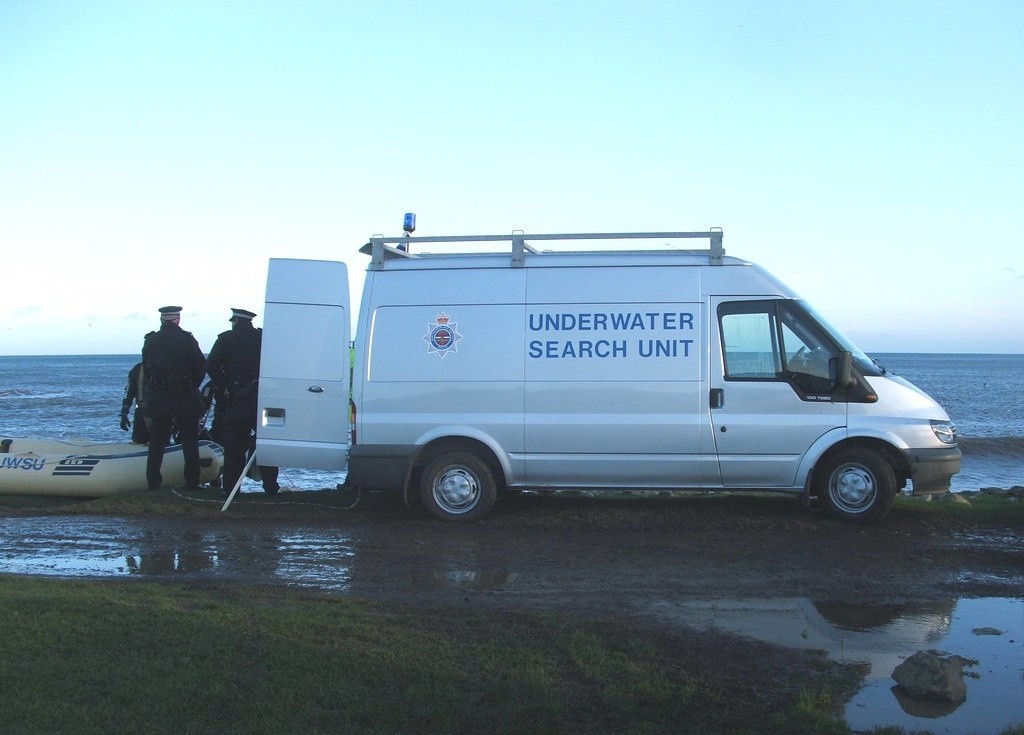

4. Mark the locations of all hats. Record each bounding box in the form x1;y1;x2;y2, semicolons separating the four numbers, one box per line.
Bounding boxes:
158;306;182;316
229;308;256;321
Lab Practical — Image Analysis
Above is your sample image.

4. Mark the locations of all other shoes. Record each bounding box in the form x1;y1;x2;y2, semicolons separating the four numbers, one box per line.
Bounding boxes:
223;490;240;497
189;482;209;489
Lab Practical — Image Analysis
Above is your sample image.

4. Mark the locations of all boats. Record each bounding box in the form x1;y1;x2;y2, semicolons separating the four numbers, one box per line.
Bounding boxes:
0;438;225;497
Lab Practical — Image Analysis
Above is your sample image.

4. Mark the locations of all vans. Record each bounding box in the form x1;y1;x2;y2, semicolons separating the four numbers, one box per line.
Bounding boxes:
255;213;962;525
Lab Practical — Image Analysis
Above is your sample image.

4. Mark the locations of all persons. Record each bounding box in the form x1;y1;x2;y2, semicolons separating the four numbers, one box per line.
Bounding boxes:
119;305;280;498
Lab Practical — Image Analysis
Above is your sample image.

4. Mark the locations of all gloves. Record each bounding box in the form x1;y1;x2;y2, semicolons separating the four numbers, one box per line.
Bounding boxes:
120;414;131;432
